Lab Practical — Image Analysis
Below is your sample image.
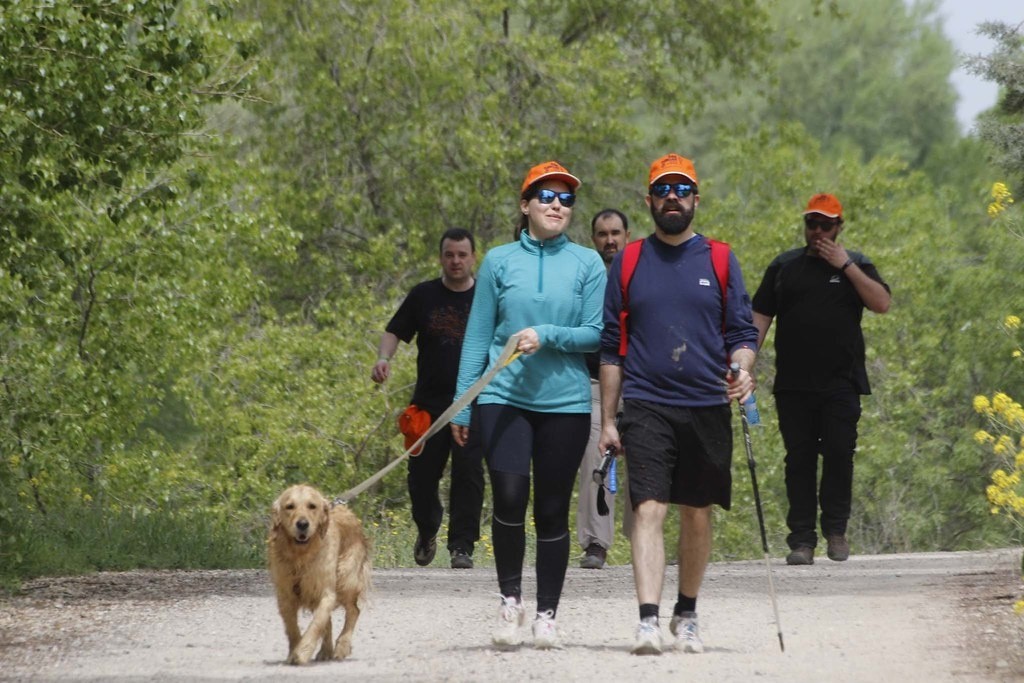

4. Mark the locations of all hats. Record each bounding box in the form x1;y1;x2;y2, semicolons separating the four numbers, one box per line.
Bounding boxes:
804;195;842;218
649;153;698;191
521;161;581;193
400;405;429;456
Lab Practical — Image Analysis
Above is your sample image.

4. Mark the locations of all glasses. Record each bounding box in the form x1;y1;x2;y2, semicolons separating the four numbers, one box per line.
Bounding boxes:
805;217;839;232
650;183;697;188
528;189;577;207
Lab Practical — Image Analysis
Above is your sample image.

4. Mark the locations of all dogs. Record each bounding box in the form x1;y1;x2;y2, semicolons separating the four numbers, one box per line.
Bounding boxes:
267;485;370;667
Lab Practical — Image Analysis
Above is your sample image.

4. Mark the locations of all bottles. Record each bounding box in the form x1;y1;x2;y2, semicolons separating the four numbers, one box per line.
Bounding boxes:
745;394;760;424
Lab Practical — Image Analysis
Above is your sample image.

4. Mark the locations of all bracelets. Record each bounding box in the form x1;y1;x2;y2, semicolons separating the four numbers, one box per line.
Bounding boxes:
379;356;390;361
841;259;853;271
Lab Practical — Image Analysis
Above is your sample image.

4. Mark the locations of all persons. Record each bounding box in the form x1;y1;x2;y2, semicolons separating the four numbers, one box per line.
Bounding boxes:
751;194;892;565
450;161;608;649
597;154;760;654
576;209;631;568
371;228;486;569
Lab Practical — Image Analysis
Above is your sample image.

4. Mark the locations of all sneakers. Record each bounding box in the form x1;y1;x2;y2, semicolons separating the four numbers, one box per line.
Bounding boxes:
413;532;436;566
669;611;703;654
491;592;526;645
828;534;849;560
631;615;661;655
451;547;473;568
532;610;561;649
787;546;814;565
580;543;606;569
